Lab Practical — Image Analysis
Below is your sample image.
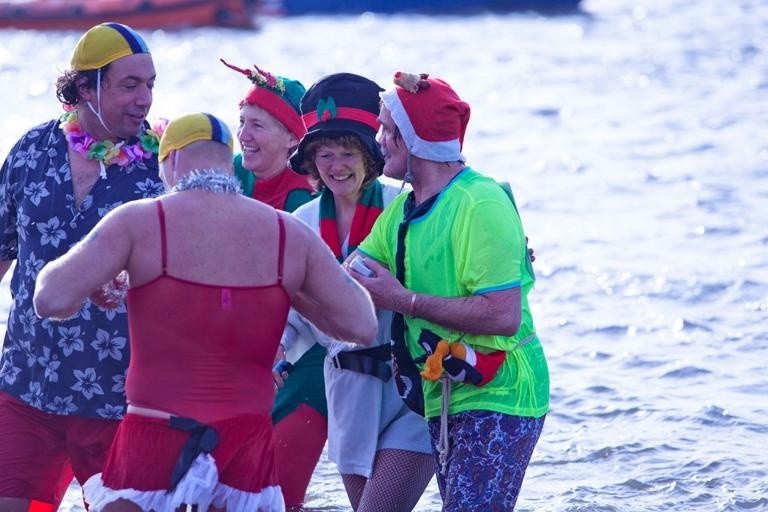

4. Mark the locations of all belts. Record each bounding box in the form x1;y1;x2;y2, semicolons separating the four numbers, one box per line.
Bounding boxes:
329;342;391;382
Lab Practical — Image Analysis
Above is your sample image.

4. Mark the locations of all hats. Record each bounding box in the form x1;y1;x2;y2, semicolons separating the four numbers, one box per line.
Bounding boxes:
286;73;385;176
239;77;305;140
380;72;470;163
158;112;232;164
70;22;152;71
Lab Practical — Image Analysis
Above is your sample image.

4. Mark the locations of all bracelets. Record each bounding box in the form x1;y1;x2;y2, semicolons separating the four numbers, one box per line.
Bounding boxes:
409;293;417;315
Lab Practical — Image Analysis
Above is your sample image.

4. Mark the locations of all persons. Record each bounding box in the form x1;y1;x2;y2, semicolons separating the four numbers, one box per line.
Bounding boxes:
341;73;549;511
219;53;323;213
0;20;165;512
32;114;378;512
273;72;535;511
